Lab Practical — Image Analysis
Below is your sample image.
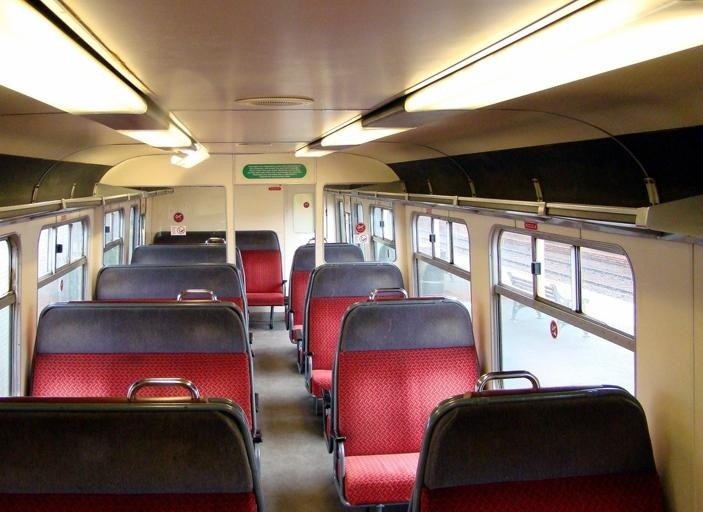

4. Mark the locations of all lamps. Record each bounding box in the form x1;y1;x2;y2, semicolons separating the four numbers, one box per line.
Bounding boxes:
293;1;702;158
0;1;212;171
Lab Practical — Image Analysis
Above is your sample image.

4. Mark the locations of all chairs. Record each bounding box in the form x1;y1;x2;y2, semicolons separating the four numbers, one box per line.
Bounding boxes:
408;370;667;512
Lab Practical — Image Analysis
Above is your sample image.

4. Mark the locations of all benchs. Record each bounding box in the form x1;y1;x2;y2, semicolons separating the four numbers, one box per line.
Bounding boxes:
288;238;482;511
509;272;589;338
153;230;287;328
1;242;264;512
